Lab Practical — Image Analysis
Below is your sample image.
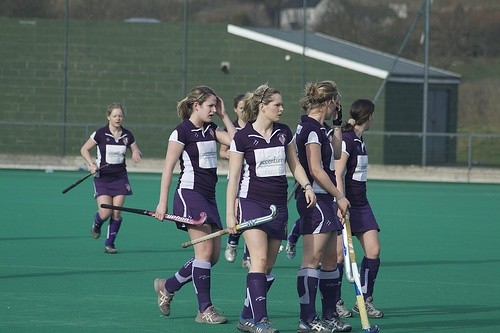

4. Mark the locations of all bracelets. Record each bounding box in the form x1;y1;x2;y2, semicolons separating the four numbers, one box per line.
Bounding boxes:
89;162;95;166
336;194;345;201
301;184;311;193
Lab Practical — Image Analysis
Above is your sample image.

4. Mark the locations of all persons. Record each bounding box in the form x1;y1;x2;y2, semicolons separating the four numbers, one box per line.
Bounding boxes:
154;81;383;333
80;103;142;254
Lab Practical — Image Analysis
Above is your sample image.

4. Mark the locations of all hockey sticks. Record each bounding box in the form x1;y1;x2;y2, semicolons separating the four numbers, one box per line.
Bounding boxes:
181;204;279;248
101;204;208;227
287;182;300;202
344;208;380;333
342;223;360;283
62;164;109;193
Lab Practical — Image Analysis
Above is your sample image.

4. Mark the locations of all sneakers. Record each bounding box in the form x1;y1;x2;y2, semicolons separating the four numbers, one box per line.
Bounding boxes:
195;305;228;324
105;246;117;253
335;299;353;318
286;242;296;258
297;314;337;333
225;243;237;262
252;317;280;333
352;296;384;318
237;315;255;331
154;278;174;316
321;316;352;333
92;223;101;238
242;257;251;267
